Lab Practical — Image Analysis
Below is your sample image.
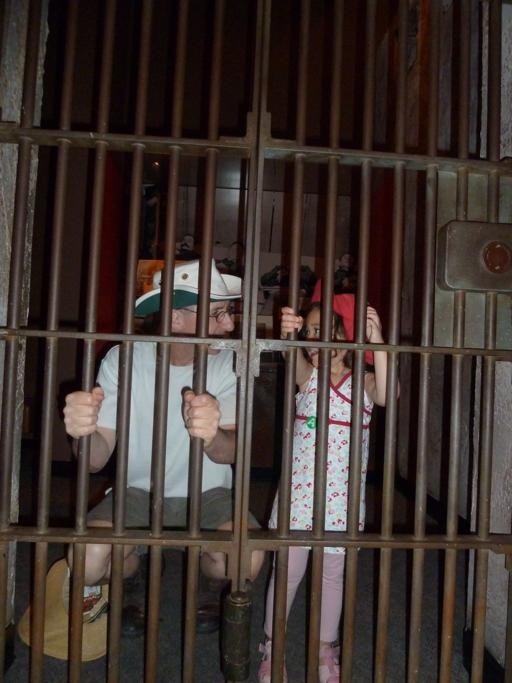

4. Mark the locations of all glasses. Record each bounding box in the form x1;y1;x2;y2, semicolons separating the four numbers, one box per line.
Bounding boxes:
176;301;237;323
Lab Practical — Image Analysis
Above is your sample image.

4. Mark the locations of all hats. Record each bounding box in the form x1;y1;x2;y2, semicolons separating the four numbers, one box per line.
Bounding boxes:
134;258;244;319
310;278;374;367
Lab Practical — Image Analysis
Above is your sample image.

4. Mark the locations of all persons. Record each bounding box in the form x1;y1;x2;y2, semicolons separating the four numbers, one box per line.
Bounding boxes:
257;280;403;683
63;257;267;639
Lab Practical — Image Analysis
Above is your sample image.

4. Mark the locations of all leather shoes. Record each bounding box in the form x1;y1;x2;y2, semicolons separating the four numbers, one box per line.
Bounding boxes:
197;576;229;634
121;552;165;638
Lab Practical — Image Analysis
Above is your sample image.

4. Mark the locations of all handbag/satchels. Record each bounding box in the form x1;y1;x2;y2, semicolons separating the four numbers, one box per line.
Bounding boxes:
19;559;108;663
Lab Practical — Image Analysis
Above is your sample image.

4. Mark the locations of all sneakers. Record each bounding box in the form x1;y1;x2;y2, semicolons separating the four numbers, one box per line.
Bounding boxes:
319;644;340;682
259;639;288;683
